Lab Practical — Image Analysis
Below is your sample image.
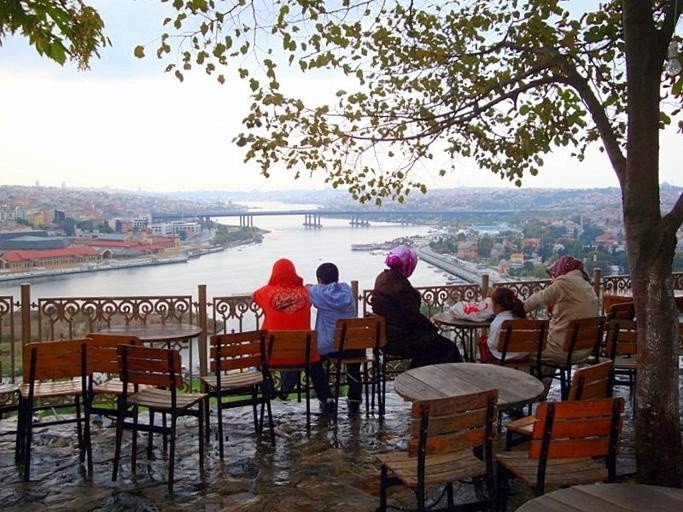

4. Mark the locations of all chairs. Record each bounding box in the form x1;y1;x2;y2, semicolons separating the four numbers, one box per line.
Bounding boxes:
317;317;385;436
495;319;551;376
492;396;625;511
14;337;94;483
83;331;168;458
604;291;683;404
201;328;276;459
112;343;210;494
533;315;606;401
504;360;617;450
393;363;545;448
250;329;320;435
373;387;498;509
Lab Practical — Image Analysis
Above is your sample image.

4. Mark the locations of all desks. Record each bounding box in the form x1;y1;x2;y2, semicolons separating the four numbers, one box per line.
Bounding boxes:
434;309;496;364
96;323;205;456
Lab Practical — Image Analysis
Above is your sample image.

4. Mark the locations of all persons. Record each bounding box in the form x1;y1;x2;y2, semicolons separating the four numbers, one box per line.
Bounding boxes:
250;259;319;400
521;256;600;403
477;285;530;367
304;262;363;415
371;245;464;367
